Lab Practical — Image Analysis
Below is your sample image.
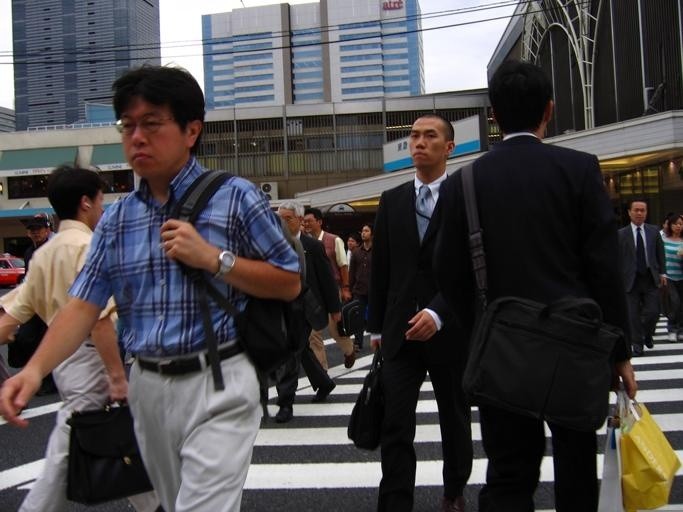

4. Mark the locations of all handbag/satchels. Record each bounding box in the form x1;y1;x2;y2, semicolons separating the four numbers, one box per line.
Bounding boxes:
598;384;682;512
346;339;394;452
335;299;364;335
65;392;155;505
457;295;634;428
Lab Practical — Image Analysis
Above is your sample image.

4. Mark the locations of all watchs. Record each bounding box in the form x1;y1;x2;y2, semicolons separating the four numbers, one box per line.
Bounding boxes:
214;247;237;280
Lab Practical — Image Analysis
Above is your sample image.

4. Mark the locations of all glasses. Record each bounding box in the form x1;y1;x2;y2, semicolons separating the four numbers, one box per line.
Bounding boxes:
116;115;176;136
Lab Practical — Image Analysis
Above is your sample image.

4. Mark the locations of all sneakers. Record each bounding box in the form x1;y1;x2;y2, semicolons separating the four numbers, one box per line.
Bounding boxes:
345;338;366;367
273;404;302;425
309;381;337;402
441;496;467;512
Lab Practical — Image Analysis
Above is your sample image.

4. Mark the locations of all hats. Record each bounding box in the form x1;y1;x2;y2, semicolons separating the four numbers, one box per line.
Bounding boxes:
19;212;51;227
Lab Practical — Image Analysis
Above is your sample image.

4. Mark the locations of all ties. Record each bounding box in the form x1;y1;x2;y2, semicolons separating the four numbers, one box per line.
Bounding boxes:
634;225;652;288
413;188;436;239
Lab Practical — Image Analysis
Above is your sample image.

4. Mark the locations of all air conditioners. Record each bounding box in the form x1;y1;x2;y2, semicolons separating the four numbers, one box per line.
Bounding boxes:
260;181;279;200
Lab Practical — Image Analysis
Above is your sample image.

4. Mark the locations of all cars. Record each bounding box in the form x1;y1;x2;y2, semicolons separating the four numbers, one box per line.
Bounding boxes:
0;253;25;286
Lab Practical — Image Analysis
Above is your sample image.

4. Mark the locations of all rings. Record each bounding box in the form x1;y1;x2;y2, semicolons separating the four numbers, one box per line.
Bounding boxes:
163;241;171;250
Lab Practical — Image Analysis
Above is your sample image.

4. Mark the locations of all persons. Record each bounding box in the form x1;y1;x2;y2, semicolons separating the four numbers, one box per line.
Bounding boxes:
618;201;683;351
364;115;476;511
273;201;374;420
433;61;638;512
11;212;60;398
1;65;301;512
1;164;130;512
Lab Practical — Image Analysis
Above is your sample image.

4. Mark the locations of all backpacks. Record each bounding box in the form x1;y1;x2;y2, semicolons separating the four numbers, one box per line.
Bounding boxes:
164;169;338;393
